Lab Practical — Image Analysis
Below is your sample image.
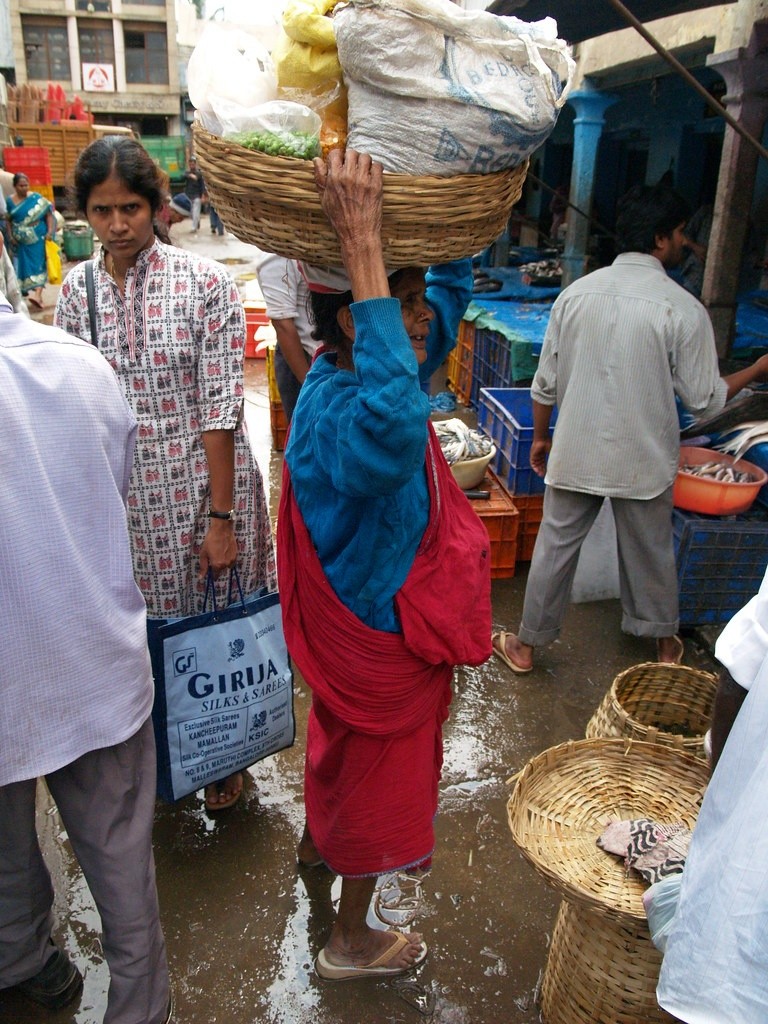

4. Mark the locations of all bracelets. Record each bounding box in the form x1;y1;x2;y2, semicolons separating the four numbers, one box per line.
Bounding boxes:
47;233;52;238
9;237;13;241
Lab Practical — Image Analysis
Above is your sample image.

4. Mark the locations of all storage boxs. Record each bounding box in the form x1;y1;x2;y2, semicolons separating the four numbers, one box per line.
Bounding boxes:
448;314;768;626
243;312;289;451
2;146;56;210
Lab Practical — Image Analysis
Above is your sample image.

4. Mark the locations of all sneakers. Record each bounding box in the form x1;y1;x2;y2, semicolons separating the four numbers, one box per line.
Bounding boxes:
9;936;83;1011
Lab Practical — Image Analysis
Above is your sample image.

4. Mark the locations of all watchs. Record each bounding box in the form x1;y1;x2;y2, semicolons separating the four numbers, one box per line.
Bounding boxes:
207;509;236;521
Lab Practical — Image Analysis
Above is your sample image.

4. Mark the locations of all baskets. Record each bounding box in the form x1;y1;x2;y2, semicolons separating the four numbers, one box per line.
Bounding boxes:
502;737;715;927
190;117;530;269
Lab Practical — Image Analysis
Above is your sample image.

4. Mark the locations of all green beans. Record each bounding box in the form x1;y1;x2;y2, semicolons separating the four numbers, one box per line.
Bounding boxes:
223;130;320;161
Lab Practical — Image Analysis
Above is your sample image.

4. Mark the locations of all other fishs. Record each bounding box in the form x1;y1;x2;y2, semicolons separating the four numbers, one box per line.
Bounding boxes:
431;418;492;465
517;259;564;278
681;462;758;483
472;272;504;294
712;418;768;465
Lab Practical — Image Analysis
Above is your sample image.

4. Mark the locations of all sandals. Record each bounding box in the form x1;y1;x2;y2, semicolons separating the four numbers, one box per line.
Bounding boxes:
205;770;245;810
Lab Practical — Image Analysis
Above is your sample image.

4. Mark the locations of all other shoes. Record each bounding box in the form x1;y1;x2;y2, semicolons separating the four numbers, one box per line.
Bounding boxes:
29;297;44;309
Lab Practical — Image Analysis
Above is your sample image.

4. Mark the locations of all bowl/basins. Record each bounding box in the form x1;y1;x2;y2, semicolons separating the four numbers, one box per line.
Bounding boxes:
447;445;497;490
673;446;768;516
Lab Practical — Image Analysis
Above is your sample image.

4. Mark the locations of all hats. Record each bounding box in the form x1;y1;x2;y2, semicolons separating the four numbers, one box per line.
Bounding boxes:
168;193;192;217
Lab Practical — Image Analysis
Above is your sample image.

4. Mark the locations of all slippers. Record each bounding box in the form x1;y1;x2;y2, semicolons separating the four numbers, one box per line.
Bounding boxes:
655;635;684;664
491;630;534;673
299;860;324;867
315;930;428;980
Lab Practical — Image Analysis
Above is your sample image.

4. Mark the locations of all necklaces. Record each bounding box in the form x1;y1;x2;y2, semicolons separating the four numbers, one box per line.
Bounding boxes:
110;259;114;278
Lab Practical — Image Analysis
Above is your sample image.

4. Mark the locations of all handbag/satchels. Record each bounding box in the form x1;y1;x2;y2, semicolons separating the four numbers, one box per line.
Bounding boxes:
146;562;296;804
45;239;63;285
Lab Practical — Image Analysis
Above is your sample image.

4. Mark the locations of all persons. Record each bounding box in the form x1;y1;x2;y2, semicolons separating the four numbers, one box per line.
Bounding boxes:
275;150;491;979
548;184;597;238
492;188;768;673
257;252;325;424
656;560;768;1024
0;228;166;1024
52;135;276;809
183;156;206;234
678;203;768;289
210;206;224;236
5;172;55;311
158;193;191;229
